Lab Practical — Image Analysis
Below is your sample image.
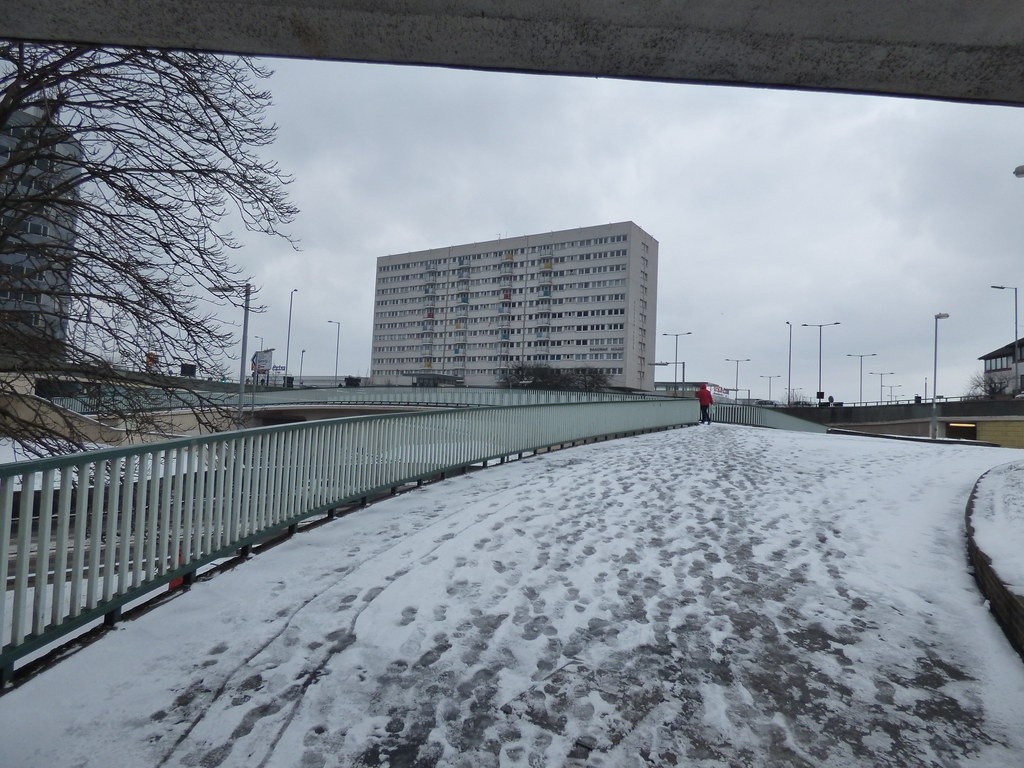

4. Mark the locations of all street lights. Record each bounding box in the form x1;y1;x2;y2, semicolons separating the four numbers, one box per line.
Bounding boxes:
990;286;1019;399
802;322;841;407
328;320;340;387
255;336;263;351
932;313;950;402
760;375;781;405
663;331;692;398
846;353;877;406
869;372;904;406
725;359;752;404
283;289;298;388
786;321;792;405
299;350;305;387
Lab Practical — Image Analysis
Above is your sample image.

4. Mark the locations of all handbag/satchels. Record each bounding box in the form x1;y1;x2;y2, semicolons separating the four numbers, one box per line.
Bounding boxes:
710;405;715;413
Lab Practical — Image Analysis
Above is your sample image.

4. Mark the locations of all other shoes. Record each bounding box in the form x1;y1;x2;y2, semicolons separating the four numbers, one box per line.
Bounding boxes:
708;420;711;425
699;421;704;424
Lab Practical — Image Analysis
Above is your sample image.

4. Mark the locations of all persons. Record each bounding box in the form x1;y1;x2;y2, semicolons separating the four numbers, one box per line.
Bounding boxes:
695;384;714;425
